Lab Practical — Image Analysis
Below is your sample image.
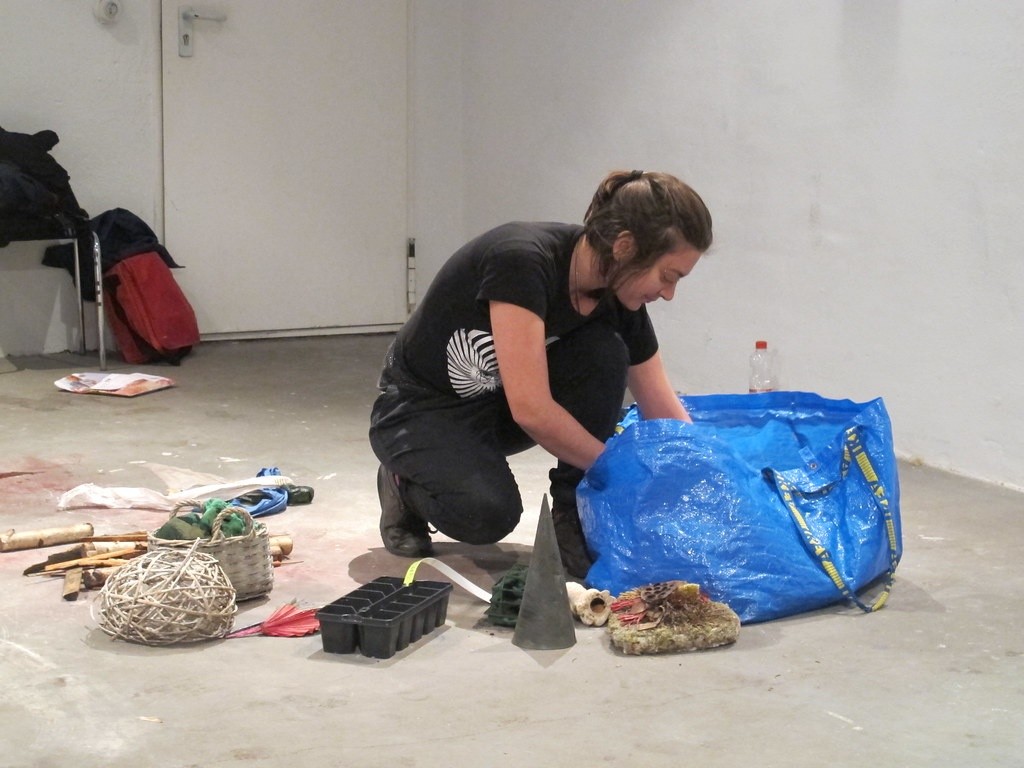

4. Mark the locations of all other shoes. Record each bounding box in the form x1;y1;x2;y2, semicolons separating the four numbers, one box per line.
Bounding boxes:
378;462;439;558
552;505;592;579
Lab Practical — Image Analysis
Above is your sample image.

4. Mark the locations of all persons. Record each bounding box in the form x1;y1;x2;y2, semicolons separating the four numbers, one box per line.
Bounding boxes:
367;170;714;558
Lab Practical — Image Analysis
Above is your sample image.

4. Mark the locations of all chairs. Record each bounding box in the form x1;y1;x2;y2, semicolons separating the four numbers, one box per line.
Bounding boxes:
0;229;107;371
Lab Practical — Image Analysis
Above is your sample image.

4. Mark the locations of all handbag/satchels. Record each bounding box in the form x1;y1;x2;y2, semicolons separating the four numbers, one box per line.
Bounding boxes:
577;392;901;625
1;123;90;245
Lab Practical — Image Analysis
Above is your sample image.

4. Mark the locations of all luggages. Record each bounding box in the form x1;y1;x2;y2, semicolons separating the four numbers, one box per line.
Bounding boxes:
104;251;200;365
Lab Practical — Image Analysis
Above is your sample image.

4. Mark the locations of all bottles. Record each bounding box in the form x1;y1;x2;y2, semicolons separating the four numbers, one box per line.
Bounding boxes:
749;341;774;394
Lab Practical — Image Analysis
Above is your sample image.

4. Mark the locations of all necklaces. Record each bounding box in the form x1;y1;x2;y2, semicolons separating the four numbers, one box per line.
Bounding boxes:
575;243;582;316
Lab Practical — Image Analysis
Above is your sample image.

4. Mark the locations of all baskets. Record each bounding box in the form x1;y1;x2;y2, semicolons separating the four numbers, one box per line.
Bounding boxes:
146;498;273;602
